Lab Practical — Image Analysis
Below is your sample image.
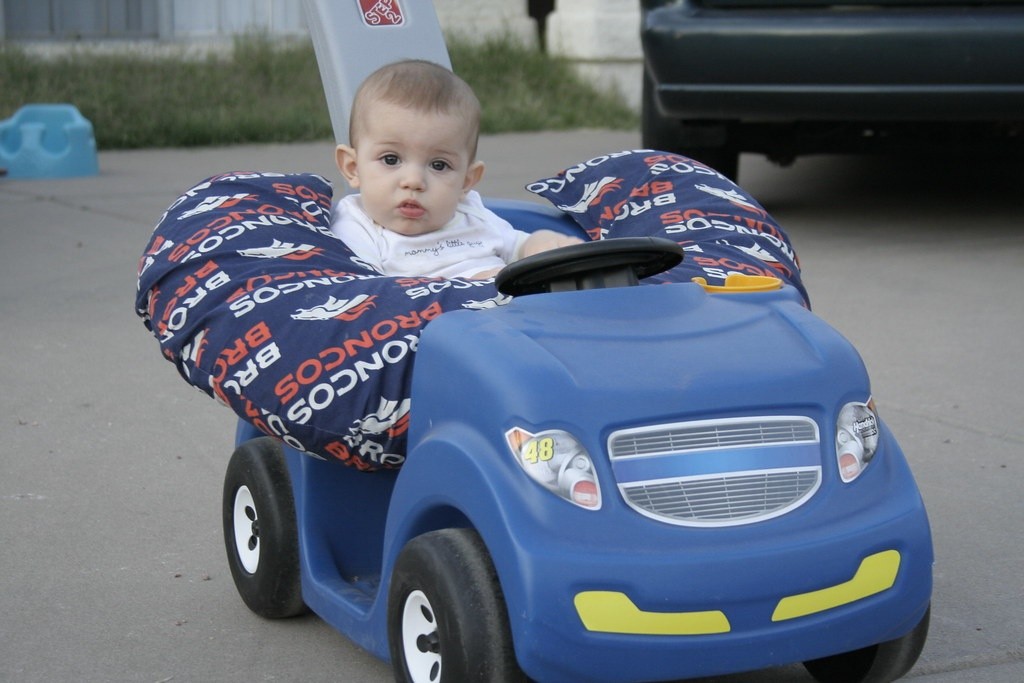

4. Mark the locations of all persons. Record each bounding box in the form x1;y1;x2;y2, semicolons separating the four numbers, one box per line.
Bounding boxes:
333;59;584;280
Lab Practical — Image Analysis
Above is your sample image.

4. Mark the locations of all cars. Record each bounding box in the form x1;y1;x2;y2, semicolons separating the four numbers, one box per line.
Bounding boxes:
636;0;1023;195
223;234;936;683
